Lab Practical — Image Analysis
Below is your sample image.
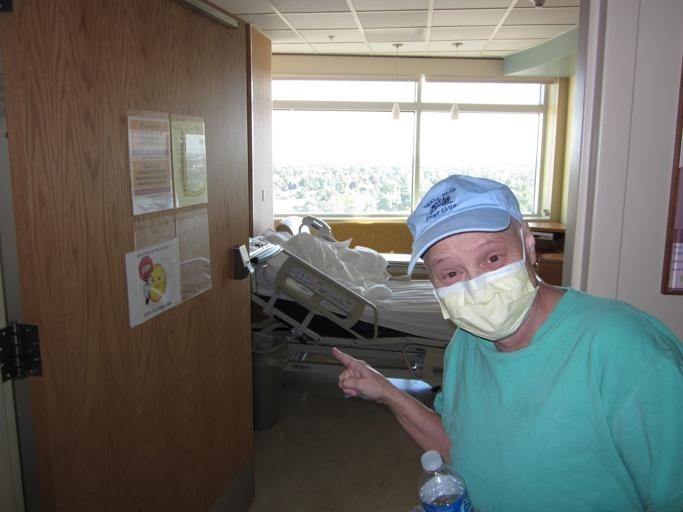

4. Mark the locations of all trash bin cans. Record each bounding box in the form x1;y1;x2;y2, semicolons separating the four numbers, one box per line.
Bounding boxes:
251;331;288;430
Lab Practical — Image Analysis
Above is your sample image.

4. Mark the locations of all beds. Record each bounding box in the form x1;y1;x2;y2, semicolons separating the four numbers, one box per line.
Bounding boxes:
251;223;457;360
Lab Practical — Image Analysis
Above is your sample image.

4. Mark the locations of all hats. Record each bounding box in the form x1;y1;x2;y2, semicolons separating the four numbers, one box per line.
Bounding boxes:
404;172;524;281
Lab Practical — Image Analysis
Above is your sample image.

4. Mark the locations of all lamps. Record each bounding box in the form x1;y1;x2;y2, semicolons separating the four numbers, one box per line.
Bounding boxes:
451;41;462;122
391;42;407;119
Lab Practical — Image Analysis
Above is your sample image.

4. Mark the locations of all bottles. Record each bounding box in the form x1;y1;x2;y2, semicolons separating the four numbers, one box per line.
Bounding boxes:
417;449;473;512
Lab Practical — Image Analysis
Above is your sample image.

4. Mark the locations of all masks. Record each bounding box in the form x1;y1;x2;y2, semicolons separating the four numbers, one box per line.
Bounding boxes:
430;229;540;343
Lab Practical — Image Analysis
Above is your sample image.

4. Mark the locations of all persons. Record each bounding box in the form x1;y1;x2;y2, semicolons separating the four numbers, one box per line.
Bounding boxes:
331;174;682;511
